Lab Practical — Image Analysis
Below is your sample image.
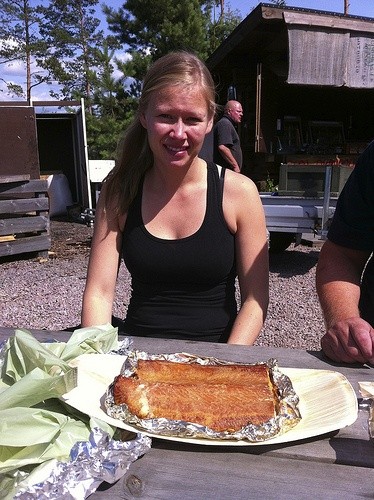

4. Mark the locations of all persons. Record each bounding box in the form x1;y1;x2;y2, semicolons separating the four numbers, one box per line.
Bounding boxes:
315;142;374;366
80;49;270;345
212;98;244;173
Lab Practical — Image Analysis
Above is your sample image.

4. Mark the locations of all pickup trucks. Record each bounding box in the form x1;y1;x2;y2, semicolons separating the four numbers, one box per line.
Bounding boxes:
256;160;354;257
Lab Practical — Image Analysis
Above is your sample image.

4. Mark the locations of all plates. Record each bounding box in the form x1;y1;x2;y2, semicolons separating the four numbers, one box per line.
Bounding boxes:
57;353;360;447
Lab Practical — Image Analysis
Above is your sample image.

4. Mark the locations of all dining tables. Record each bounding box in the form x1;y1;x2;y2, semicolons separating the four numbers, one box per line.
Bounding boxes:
0;324;374;500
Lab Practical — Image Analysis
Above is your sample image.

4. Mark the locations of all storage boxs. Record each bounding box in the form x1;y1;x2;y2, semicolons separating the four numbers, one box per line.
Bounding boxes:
278;162;355;197
